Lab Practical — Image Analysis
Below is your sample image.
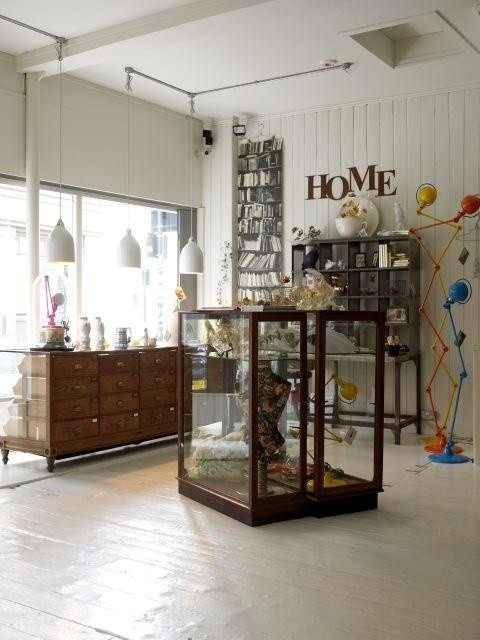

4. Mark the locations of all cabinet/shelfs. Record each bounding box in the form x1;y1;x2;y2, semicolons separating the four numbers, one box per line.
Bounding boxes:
175;310;310;526
0;344;198;473
268;310;387;518
292;238;421;445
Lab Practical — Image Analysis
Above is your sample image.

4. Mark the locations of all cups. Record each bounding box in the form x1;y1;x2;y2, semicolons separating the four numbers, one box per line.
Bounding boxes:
115;328;132;349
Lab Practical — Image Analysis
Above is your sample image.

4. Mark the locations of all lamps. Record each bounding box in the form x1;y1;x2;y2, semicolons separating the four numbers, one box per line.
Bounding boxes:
288;374;358;488
117;72;141;268
179;96;203;274
414;183;461;444
427;278;472;464
408;194;480;454
47;42;75;262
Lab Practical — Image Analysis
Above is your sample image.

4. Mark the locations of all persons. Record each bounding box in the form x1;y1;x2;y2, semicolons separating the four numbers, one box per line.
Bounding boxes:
234;362;291;461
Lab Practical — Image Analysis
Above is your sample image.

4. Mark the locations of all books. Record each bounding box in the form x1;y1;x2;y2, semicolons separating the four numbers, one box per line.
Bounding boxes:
237;188;279;203
238;252;279;270
238;288;280;304
239;306;265;313
238;219;282;235
238;153;282;171
236;235;282;253
392;230;409;236
237;272;280;287
379;243;409;268
236;170;279;188
238;204;281;219
237;135;283;155
